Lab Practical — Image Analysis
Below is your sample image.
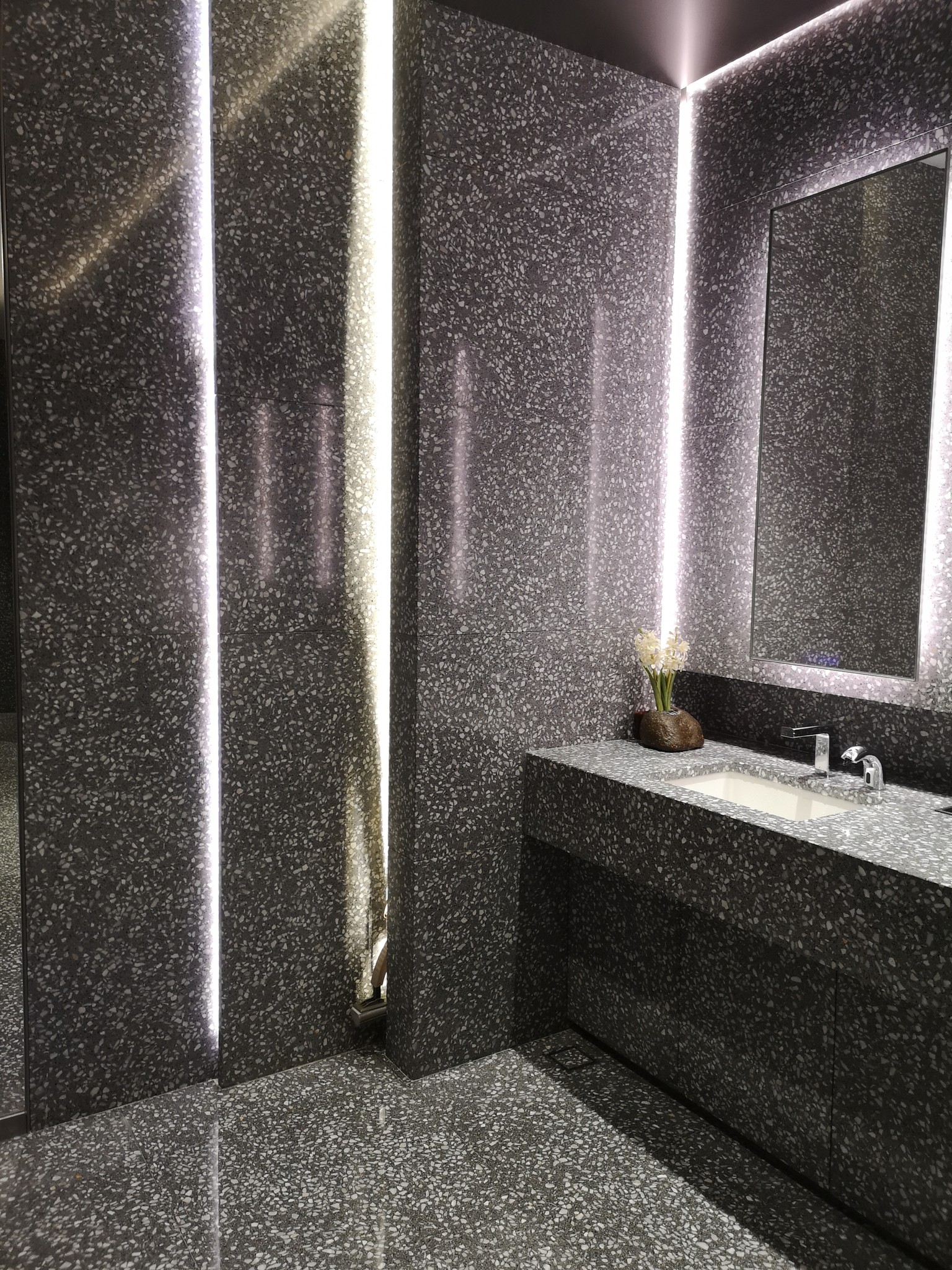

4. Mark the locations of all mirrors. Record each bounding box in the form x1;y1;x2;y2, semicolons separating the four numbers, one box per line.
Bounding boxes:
748;147;950;680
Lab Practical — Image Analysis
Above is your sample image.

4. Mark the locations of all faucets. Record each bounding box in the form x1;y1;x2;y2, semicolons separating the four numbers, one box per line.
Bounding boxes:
780;722;833;776
842;745;887;790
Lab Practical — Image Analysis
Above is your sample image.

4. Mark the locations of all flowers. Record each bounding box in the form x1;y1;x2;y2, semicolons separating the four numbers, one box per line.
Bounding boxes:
634;626;690;713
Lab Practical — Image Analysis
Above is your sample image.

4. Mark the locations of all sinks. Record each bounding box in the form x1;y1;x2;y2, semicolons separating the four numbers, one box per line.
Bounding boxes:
644;760;884;823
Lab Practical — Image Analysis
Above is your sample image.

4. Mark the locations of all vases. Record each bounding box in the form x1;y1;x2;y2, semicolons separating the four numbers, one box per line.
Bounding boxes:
640;707;704;752
